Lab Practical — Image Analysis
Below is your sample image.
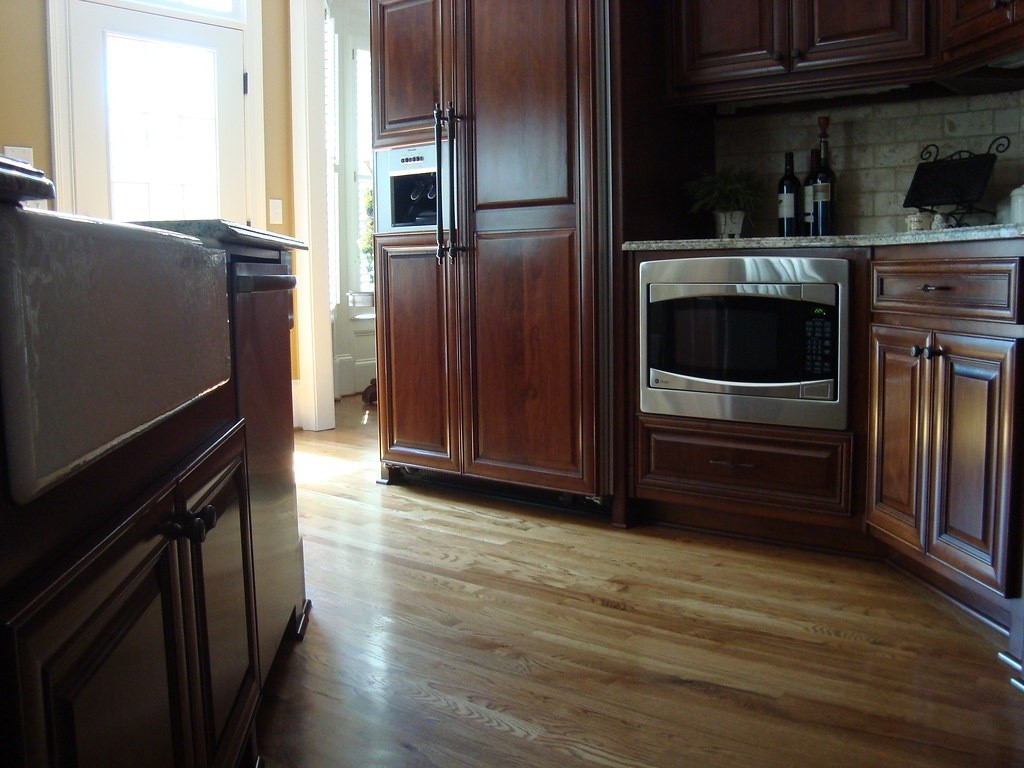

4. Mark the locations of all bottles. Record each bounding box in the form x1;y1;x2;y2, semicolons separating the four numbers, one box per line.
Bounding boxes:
776;151;802;237
812;115;836;235
801;147;817;236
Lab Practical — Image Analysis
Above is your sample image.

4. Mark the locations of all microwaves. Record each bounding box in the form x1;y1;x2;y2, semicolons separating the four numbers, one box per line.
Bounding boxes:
372;138;459;235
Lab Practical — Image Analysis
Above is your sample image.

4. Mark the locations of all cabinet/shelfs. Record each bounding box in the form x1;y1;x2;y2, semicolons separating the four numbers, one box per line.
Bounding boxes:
628;412;869;563
931;0;1023;87
662;0;940;117
1;157;312;768
868;240;1024;692
370;0;717;531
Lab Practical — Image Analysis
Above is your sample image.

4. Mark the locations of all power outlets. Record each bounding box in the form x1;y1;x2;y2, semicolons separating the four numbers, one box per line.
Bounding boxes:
4;146;34;168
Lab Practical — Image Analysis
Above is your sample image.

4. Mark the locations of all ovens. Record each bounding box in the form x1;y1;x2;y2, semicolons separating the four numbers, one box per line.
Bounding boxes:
229;252;308;696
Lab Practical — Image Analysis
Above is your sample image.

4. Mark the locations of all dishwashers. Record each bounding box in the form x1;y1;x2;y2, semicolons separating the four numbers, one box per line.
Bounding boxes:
637;257;848;430
